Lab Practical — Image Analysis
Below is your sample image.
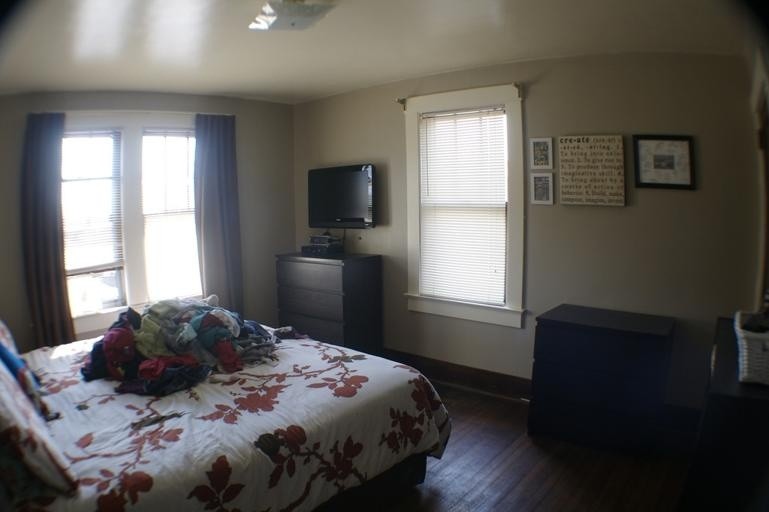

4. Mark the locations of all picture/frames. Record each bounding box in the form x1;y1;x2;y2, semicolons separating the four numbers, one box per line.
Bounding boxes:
633;135;696;191
530;137;554;205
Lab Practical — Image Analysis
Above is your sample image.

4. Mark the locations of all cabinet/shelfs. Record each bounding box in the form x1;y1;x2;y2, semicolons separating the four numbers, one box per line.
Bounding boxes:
528;304;677;457
680;316;768;510
275;253;382;356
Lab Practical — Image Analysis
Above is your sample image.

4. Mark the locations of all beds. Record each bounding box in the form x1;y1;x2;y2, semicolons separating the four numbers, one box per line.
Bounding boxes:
0;324;427;511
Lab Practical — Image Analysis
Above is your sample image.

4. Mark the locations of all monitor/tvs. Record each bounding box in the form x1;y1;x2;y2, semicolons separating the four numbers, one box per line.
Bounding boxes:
308;162;377;230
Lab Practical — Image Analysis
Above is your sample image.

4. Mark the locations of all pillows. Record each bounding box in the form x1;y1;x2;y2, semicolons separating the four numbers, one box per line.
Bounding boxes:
0;317;80;497
